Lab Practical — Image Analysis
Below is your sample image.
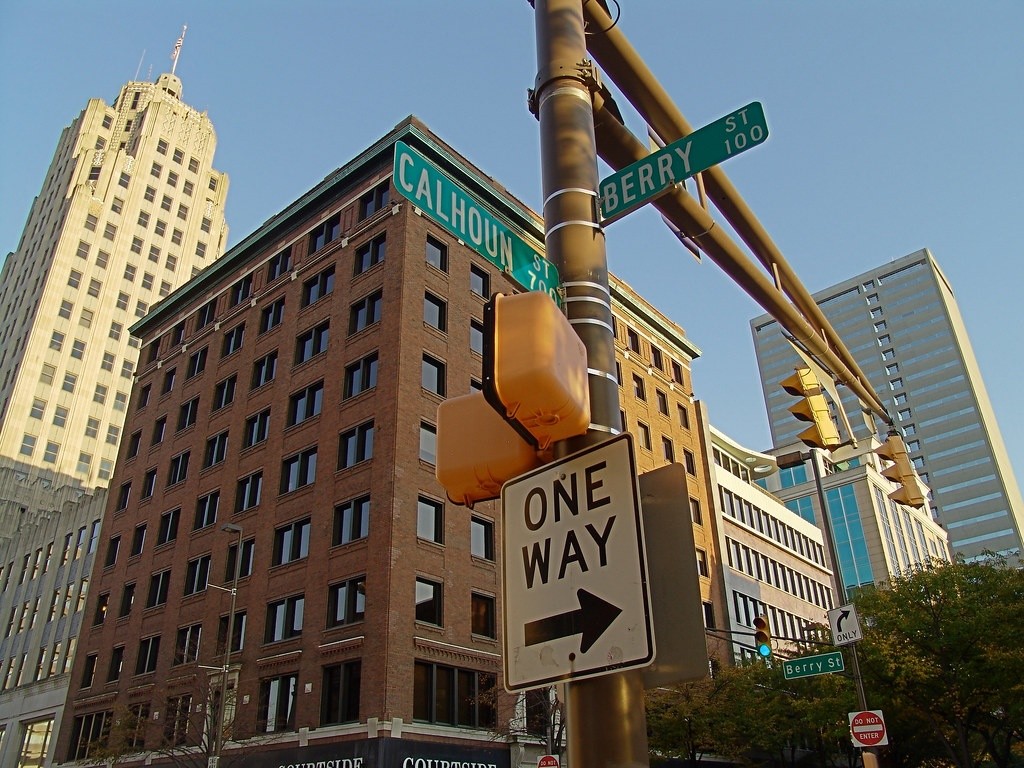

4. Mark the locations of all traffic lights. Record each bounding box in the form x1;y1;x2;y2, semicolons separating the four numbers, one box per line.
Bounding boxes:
869;432;926;511
479;291;591;452
779;367;844;451
753;615;774;659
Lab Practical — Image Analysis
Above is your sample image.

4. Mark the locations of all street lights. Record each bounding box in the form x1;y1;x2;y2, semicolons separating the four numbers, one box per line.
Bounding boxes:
213;523;245;767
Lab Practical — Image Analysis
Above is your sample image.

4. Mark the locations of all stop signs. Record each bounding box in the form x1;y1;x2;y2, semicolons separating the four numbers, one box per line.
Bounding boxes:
848;710;890;747
537;755;560;768
